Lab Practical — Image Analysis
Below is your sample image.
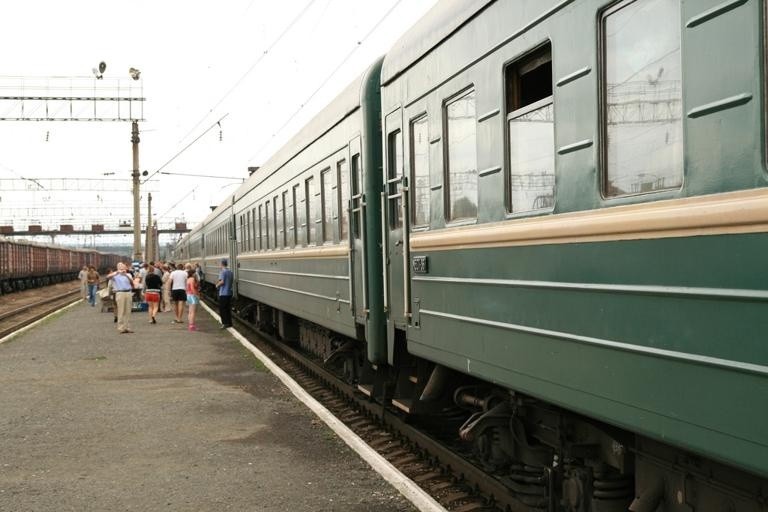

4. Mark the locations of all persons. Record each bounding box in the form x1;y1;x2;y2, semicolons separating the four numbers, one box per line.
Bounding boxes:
216;258;233;329
78;261;205;333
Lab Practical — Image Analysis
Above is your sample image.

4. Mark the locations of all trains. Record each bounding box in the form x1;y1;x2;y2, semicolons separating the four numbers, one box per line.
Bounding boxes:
162;0;766;512
0;238;132;294
